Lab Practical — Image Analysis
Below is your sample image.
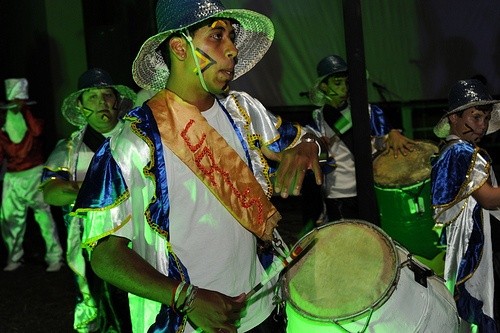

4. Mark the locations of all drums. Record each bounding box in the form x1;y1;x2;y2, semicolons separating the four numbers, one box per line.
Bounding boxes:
281;219;460;333
372;140;447;279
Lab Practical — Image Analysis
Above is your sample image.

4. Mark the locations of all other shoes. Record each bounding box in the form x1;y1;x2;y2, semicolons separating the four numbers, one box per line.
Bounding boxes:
47;260;64;271
3;261;21;271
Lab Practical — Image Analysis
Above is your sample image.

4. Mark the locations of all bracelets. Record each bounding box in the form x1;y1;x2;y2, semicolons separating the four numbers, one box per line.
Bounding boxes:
171;279;199;315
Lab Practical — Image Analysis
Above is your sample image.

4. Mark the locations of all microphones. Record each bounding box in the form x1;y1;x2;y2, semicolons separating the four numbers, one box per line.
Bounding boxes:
300;92;309;96
373;83;385;90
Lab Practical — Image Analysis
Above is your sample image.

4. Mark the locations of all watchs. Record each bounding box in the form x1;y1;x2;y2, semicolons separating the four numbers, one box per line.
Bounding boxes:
299;132;323;158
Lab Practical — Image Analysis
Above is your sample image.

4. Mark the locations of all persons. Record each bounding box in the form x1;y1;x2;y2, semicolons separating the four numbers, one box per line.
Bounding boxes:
66;0;330;333
426;71;500;333
0;76;63;271
306;54;417;228
39;66;137;216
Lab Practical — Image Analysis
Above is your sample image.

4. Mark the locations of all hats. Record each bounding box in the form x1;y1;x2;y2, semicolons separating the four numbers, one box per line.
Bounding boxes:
433;78;500;138
0;79;38;109
61;68;137;130
132;0;275;92
311;54;370;105
